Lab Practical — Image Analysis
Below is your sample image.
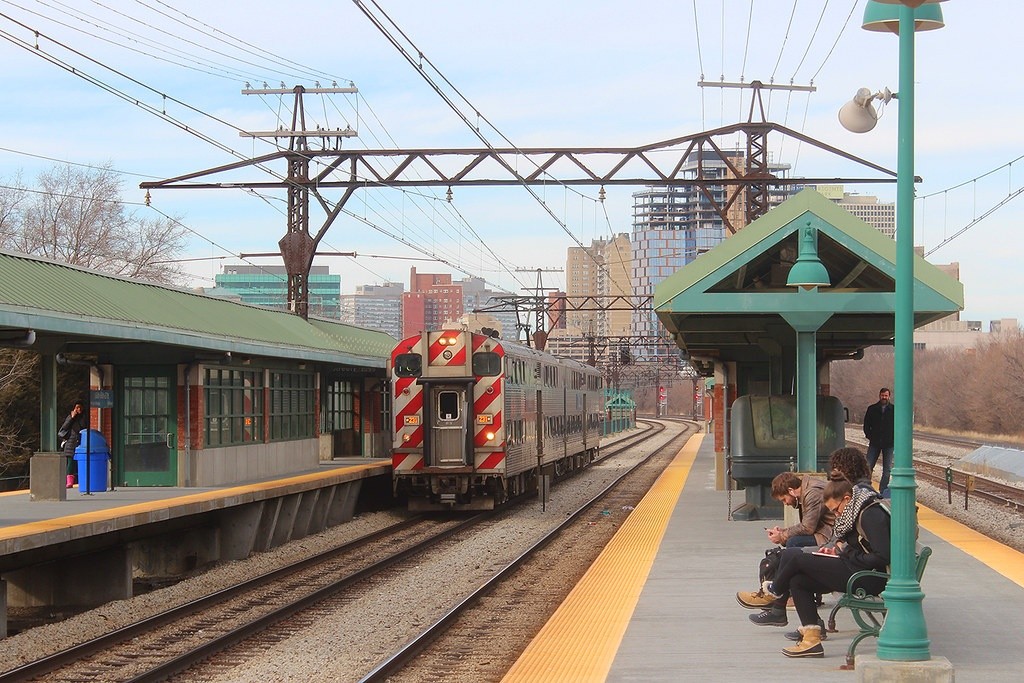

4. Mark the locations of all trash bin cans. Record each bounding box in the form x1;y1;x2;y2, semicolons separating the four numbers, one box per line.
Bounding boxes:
73;429;111;492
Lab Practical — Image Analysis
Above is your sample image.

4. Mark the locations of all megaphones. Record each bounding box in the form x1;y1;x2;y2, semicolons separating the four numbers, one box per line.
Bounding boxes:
839;87;878;133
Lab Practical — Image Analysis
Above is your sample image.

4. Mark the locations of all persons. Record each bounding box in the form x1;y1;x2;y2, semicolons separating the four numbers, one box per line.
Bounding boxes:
61;399;87;489
735;470;891;657
864;387;895;493
766;472;835;611
749;445;872;641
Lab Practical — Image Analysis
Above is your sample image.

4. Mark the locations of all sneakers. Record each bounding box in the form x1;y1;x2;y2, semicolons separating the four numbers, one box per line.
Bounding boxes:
749;610;789;627
784;620;826;640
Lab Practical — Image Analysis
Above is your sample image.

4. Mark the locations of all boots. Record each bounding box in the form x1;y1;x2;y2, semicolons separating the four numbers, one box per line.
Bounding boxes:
781;625;824;657
65;475;75;488
734;581;784;610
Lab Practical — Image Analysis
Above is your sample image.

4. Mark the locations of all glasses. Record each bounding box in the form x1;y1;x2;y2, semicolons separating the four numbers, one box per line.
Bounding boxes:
831;496;845;514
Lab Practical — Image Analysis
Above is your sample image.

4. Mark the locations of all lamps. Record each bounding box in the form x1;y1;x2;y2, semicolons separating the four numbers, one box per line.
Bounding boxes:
785;222;831;286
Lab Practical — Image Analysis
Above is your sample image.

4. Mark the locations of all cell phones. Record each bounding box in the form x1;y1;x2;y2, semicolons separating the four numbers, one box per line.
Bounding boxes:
765;527;773;535
812;551;839;558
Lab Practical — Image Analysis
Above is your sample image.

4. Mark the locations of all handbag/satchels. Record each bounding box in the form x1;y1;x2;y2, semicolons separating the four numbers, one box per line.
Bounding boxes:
58;426;70;440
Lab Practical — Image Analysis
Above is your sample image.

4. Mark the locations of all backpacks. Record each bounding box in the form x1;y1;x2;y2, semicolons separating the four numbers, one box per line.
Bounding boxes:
856;498;919;542
759;547;785;586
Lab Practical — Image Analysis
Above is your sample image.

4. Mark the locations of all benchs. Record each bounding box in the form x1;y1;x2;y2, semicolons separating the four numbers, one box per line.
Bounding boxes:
814;541;932;670
